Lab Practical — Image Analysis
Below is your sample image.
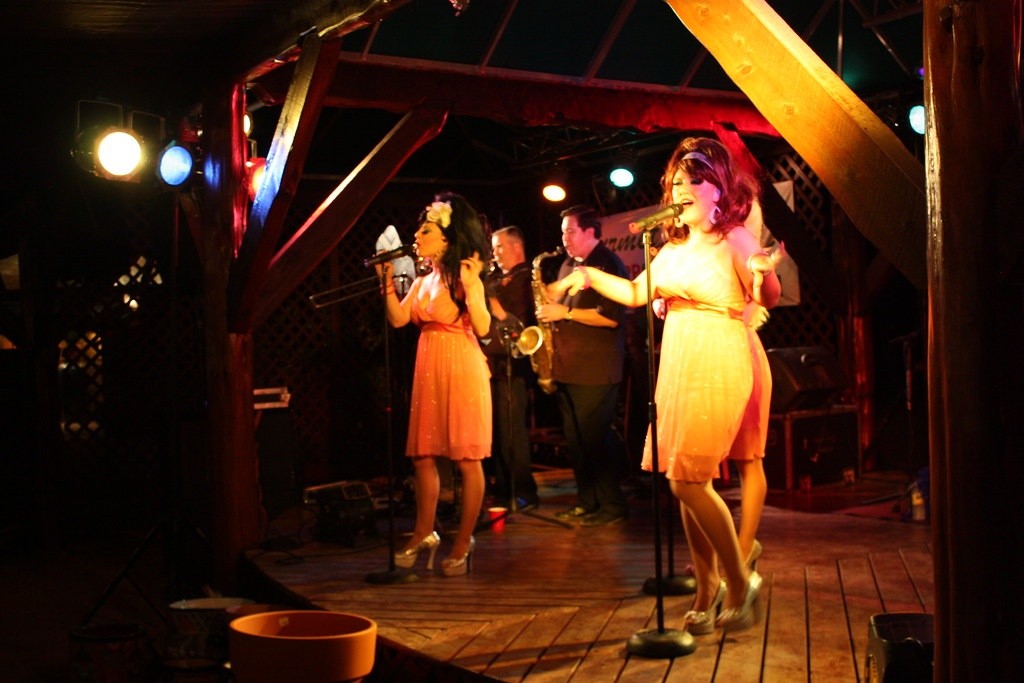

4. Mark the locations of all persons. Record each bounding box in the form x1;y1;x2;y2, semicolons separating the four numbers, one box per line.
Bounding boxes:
375;191;492;576
481;225;541;511
534;206;627;519
550;137;786;634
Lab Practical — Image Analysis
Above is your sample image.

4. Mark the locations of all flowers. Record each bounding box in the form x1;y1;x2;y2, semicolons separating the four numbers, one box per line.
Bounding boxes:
426;200;453;227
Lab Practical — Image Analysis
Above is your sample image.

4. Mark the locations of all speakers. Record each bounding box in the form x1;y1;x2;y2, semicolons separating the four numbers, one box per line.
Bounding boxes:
864;612;935;683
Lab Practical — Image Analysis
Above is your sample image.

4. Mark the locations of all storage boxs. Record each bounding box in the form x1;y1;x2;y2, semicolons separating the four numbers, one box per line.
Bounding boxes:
769;407;861;497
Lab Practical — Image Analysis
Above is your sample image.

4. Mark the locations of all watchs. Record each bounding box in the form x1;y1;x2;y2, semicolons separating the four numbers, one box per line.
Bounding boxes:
566;307;572;319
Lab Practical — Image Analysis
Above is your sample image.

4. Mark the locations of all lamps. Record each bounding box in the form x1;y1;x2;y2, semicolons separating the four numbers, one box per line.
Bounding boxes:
542;166;573;203
609;147;648;189
159;138;210;195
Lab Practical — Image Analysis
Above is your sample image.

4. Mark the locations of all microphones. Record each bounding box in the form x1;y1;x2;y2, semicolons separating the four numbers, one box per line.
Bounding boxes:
364;244;412;267
502;325;518;340
628;204;684;233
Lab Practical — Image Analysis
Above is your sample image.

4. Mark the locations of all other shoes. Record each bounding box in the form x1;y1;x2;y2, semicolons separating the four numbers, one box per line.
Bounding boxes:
505;496;536;519
580;510;625;526
553;506;599;521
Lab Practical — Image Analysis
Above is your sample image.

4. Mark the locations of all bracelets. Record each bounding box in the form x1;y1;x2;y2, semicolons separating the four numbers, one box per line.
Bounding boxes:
465;294;478;305
747;252;772;277
380;281;395;288
381;288;395;295
573;266;590;291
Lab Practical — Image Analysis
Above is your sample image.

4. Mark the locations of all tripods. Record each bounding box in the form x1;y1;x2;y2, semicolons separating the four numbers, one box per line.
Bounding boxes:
473;332;576;536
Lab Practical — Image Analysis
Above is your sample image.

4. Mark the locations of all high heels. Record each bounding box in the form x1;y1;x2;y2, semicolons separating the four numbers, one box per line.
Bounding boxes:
720;538;762;582
441;535;475;577
684;578;696;589
684;579;726;633
717;570;763;630
395;531;441;570
